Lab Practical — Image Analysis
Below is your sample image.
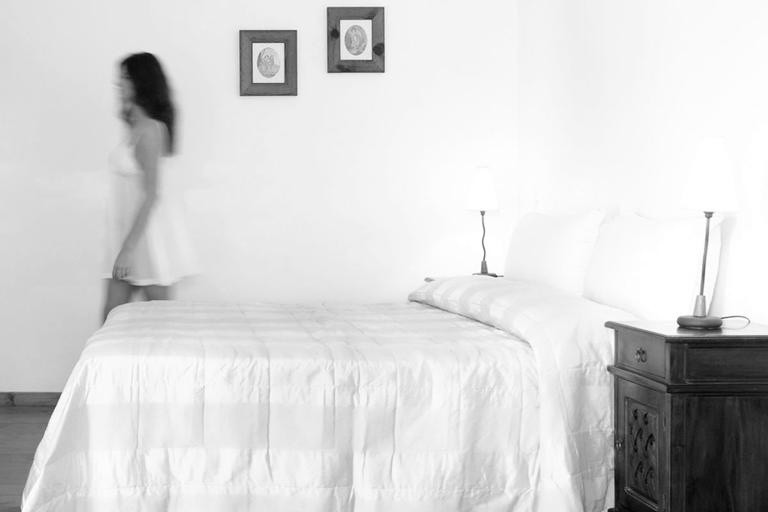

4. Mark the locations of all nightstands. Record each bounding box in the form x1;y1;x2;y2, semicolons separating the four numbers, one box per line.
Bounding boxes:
607;320;768;512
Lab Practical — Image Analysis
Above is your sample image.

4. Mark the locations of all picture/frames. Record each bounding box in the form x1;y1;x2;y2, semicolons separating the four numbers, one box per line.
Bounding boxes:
241;8;385;96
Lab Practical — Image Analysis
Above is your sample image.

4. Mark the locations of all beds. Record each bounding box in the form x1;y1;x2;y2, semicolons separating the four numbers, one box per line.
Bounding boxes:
19;192;733;510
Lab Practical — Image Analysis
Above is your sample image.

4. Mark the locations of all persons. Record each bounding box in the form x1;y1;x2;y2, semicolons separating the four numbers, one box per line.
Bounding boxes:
102;52;199;327
